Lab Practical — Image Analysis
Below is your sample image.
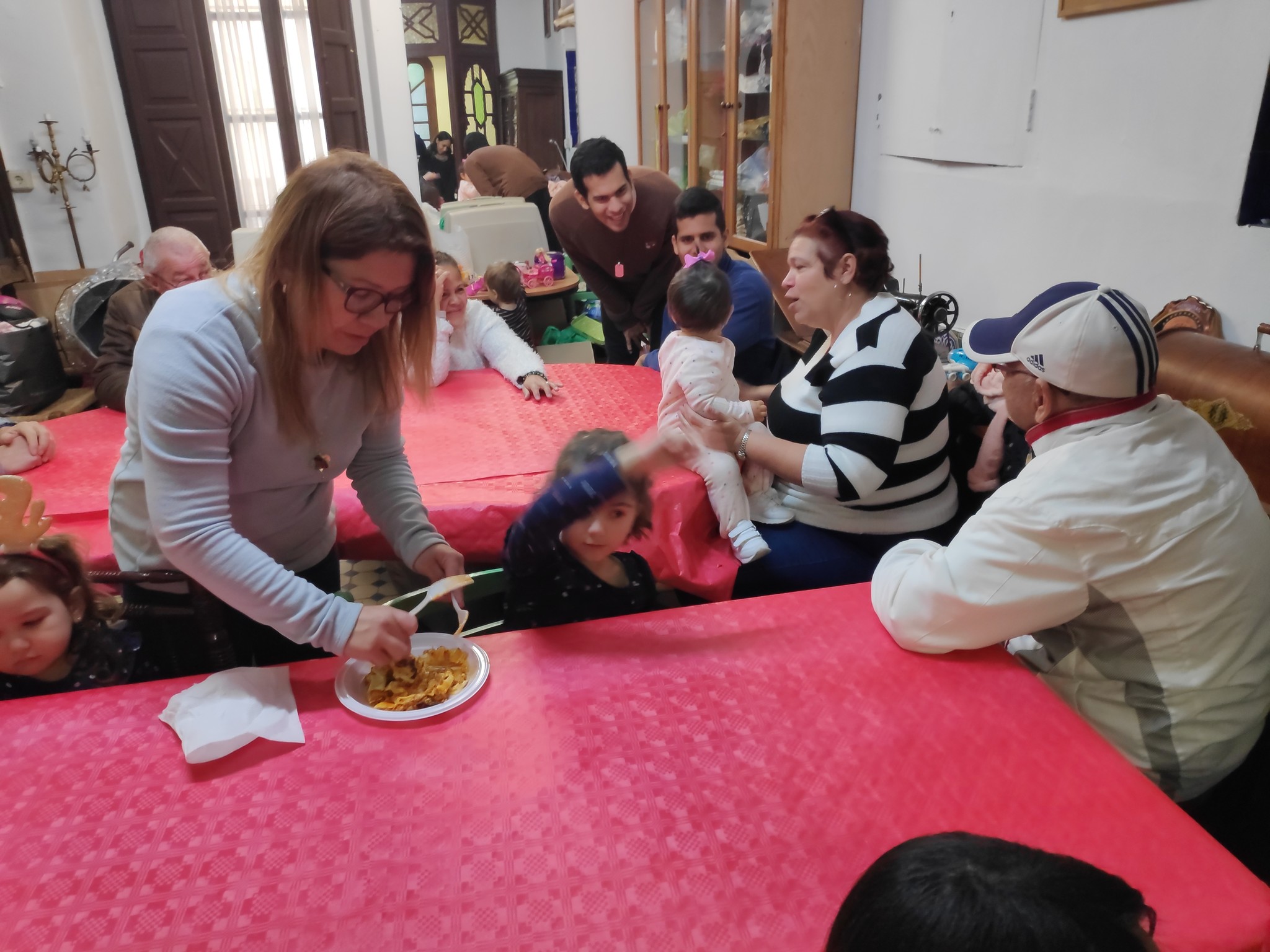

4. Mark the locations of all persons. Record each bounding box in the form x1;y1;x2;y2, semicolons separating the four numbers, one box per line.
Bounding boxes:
0;474;156;702
413;130;562;251
500;421;699;629
0;416;56;476
657;248;794;564
548;137;684;366
399;251;564;401
677;204;961;595
871;279;1270;801
635;186;779;385
91;225;221;414
107;146;465;681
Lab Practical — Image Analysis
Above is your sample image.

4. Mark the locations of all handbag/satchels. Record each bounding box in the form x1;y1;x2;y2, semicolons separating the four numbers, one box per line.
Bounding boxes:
0;317;66;418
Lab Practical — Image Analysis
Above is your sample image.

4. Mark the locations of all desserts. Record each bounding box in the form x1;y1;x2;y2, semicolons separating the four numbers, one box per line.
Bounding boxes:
364;645;469;710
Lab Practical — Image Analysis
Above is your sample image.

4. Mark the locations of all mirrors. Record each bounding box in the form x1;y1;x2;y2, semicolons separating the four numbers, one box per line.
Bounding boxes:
400;0;579;210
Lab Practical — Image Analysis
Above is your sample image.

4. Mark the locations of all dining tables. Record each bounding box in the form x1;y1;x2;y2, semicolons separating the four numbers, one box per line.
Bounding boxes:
0;583;1270;952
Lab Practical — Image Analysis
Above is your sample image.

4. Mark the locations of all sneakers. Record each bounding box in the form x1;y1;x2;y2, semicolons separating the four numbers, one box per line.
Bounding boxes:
727;519;771;564
746;487;796;524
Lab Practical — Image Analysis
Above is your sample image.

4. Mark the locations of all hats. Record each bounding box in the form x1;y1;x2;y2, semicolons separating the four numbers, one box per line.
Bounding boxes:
961;281;1161;398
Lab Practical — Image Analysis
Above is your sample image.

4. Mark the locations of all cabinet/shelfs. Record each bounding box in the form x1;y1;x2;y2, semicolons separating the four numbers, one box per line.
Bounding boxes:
635;0;864;252
500;67;567;172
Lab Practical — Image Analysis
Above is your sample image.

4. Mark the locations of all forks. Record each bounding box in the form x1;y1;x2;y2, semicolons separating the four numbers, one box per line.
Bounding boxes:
451;592;469;636
409;574;474;615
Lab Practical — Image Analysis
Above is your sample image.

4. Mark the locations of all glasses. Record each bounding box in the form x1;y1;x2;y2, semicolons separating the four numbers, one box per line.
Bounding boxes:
150;267;219;288
815;204;856;254
992;363;1073;396
320;260;415;315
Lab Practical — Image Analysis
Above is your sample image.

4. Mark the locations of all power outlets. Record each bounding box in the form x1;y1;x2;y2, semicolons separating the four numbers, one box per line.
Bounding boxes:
7;169;33;191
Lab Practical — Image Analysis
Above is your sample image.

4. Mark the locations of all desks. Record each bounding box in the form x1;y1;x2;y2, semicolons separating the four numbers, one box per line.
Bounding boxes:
0;364;741;604
467;267;580;327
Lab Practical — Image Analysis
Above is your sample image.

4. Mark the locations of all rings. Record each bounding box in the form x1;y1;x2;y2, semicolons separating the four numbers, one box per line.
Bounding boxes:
546;381;549;383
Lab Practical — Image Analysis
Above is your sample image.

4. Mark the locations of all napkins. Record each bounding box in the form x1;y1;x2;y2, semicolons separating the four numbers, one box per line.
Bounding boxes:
158;666;305;763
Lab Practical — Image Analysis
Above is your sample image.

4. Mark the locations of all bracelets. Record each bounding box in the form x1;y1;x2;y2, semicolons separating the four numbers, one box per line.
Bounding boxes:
735;429;752;461
522;371;547;381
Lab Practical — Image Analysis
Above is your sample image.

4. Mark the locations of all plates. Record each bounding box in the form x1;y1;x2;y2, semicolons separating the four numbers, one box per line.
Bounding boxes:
334;632;490;722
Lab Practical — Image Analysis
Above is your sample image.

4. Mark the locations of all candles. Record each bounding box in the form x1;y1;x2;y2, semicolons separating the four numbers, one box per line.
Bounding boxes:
44;112;51;121
82;134;91;142
30;139;38;145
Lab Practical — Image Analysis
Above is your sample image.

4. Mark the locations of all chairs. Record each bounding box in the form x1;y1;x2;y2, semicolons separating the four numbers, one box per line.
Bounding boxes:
445;203;550;276
948;362;1028;527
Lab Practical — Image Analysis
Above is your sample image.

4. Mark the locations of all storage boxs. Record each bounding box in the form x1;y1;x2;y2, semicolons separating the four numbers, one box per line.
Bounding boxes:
536;341;595;363
